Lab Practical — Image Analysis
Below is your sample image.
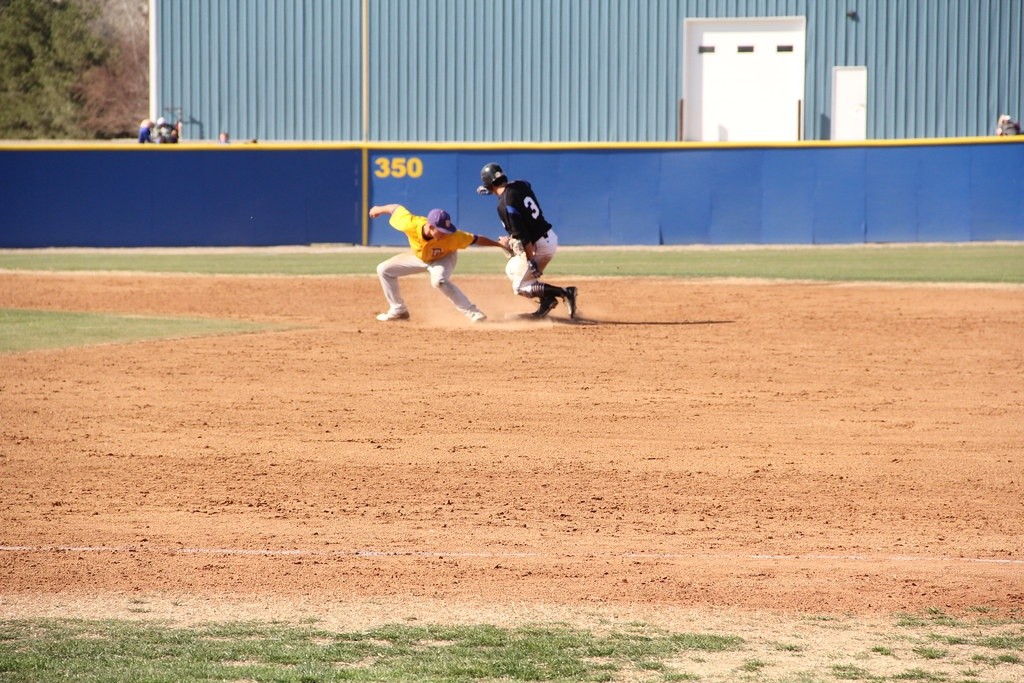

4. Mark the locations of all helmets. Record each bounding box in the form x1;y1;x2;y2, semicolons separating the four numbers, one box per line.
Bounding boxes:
480;162;503;186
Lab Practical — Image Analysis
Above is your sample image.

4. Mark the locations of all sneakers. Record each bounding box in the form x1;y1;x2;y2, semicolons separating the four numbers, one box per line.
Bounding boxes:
377;307;409;321
565;285;576;319
532;297;558;317
465;308;486;323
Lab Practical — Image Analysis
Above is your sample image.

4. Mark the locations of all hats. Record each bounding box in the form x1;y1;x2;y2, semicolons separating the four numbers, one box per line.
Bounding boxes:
427;209;456;233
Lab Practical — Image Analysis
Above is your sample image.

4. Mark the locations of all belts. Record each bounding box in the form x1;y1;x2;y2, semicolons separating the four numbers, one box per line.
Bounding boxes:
543;233;549;239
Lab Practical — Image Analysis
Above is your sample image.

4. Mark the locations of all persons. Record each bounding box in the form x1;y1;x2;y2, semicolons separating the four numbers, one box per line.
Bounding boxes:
218;133;230;143
477;163;577;318
369;204;513;322
139;118;179;143
996;115;1018;135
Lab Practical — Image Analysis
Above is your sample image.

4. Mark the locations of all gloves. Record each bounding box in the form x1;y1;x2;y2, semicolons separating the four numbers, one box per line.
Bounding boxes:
527;259;543;278
477;186;492;195
